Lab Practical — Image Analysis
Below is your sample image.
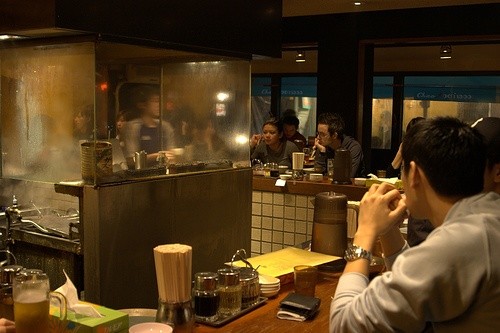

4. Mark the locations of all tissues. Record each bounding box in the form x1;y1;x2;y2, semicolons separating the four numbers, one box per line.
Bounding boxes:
366;173;402;190
48;269;129;333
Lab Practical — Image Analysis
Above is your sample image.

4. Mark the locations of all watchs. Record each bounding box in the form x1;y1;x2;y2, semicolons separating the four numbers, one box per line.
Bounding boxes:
345;246;373;262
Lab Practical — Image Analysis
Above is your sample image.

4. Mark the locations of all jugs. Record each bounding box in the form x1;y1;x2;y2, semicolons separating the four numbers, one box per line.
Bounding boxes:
331;148;352;185
309;191;359;271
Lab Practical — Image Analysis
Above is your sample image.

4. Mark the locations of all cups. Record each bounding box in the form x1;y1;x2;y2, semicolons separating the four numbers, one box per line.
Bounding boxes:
128;299;194;333
292;169;303;181
303;148;334;181
12;269;67;333
80;141;112;182
310;174;323;181
294;265;317;297
377;170;386;178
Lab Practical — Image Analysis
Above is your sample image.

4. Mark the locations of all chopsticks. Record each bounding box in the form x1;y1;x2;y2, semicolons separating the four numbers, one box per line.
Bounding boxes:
153;243;193;303
293;152;304;168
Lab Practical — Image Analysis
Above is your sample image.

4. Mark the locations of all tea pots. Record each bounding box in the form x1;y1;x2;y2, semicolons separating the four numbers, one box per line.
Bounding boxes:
252;159;265;171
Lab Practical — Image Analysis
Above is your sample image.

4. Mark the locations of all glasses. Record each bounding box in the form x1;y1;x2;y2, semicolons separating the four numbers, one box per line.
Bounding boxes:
315;131;330;138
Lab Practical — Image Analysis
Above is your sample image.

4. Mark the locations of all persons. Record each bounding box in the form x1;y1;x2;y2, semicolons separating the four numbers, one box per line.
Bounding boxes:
311;115;366;182
0;78;24;167
392;117;500;247
23;81;175;181
331;115;500;333
250;108;308;170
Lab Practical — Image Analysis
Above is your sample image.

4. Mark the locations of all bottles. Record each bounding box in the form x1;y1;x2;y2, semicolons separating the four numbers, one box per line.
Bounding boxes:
135;151;147;169
264;162;279;178
191;266;260;322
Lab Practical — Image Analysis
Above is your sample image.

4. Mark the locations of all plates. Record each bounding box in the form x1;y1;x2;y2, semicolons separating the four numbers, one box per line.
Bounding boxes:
259;276;281;297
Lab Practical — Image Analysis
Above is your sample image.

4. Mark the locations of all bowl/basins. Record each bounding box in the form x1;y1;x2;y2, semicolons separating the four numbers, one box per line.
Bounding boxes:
369;256;385;275
278;166;292;179
351;178;367;186
303;167;324;175
